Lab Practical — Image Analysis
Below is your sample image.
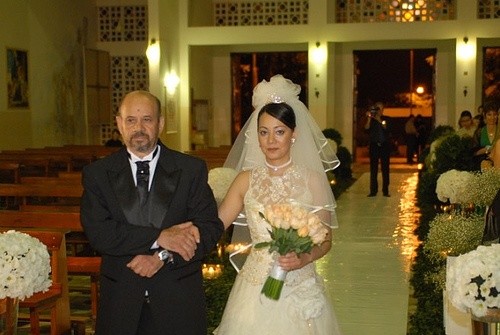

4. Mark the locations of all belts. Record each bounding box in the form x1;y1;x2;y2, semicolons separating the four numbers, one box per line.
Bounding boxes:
144;289;152;300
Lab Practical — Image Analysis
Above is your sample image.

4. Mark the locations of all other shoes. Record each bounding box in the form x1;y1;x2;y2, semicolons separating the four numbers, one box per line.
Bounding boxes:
383;193;391;197
367;192;376;197
407;159;414;163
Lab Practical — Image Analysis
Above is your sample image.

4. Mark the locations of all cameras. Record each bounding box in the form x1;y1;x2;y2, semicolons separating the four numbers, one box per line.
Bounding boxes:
369;108;379;116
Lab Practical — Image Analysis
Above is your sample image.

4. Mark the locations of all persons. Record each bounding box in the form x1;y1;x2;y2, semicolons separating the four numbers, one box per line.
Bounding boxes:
81;93;225;335
364;102;393;197
214;76;340;335
106;129;123;147
414;115;427;164
454;110;483;139
404;114;418;165
478;99;500;154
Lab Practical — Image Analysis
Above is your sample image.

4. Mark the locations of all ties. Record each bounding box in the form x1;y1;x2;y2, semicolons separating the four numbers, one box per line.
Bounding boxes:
126;146;158;208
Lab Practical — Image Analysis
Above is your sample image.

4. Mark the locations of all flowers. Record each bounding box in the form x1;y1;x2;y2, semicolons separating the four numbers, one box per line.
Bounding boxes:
253;201;328;300
0;229;53;302
435;168;475;206
207;167;238;201
445;244;500;318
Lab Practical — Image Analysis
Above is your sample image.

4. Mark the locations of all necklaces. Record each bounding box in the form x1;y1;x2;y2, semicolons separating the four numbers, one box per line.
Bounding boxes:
264;160;292;171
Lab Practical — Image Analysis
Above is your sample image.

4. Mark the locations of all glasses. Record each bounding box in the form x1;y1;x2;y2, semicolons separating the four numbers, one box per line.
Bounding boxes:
460;118;471;122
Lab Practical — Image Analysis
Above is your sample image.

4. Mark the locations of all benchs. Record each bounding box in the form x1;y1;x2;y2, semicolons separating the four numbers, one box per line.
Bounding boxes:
0;144;120;335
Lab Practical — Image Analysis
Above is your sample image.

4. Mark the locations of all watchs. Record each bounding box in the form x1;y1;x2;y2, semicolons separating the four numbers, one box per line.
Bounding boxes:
157;247;169;265
